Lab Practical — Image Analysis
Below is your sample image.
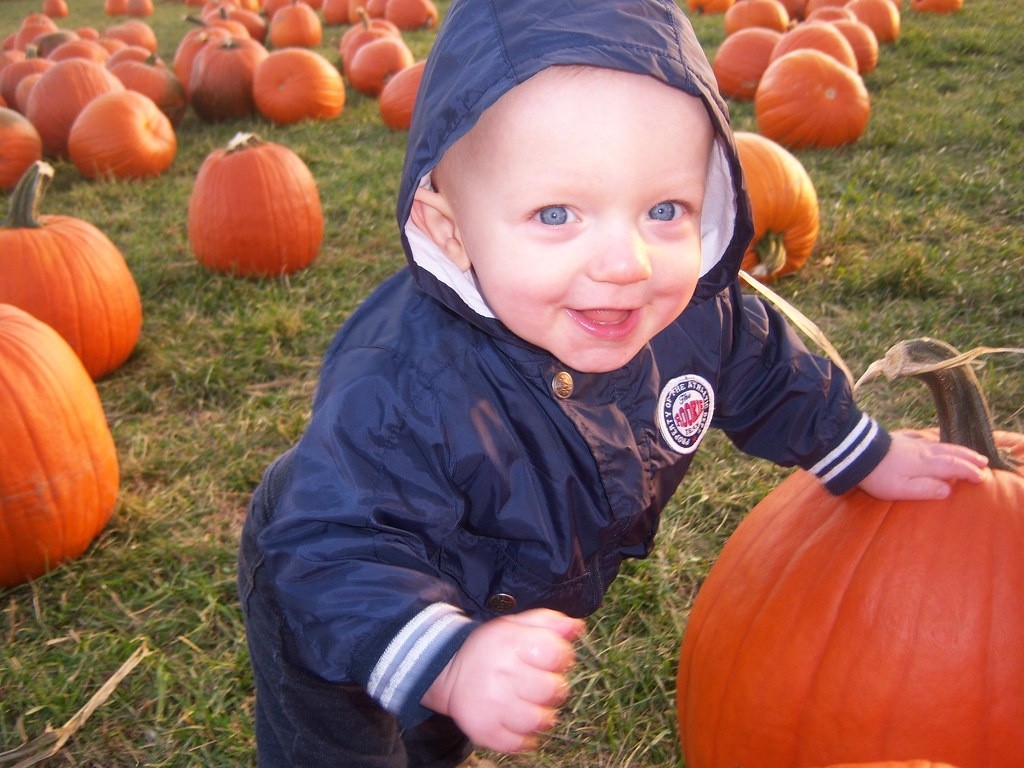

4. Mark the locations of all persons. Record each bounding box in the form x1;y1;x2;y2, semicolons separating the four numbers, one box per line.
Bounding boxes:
238;1;989;768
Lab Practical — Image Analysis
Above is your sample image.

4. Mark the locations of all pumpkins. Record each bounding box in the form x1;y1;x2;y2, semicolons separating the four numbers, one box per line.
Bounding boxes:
1;0;1024;765
